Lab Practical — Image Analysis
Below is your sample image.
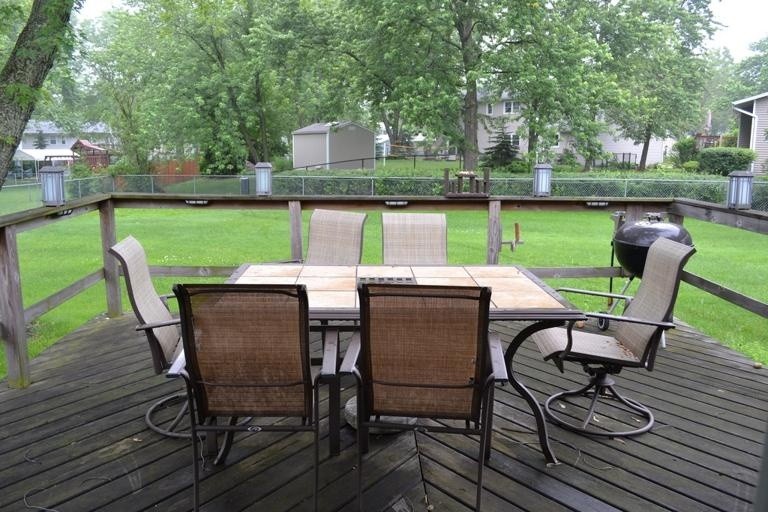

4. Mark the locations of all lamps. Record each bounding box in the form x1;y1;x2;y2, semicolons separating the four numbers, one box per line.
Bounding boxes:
534;163;553;197
38;166;66;206
728;170;755;210
255;161;272;196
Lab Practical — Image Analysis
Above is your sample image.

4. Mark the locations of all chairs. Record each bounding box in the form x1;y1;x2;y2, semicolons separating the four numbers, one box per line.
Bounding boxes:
380;213;450;267
340;281;510;512
284;209;368;365
166;283;341;512
108;233;255;443
533;236;696;438
